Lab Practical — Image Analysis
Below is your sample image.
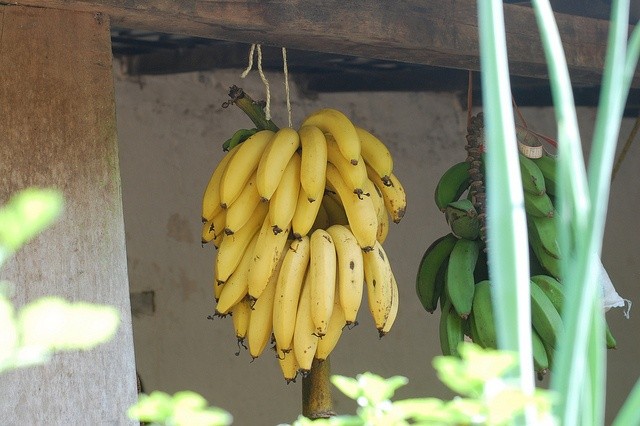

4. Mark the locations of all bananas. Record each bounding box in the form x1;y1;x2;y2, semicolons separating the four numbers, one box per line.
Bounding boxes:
202;110;406;384
416;126;619;377
